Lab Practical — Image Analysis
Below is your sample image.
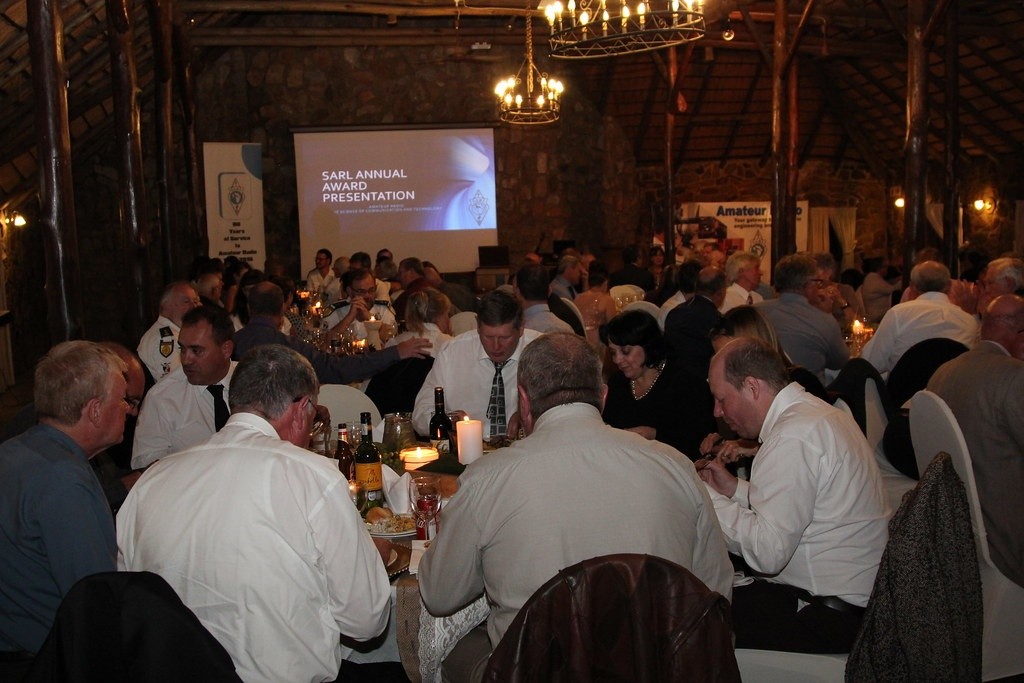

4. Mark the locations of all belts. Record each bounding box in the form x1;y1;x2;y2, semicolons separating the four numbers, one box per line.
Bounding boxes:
822;596;866;616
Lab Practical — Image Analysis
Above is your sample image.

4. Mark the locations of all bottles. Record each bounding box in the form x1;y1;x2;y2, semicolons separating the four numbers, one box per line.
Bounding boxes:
430;387;453;458
335;423;355;480
354;411;383;518
416;487;440;541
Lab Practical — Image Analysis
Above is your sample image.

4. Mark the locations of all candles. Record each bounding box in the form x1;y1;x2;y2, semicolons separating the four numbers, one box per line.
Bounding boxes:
457;415;482;464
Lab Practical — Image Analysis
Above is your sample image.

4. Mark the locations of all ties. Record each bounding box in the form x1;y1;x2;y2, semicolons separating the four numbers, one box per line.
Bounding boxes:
568;286;575;300
486;360;510;441
206;384;229;433
748;296;752;305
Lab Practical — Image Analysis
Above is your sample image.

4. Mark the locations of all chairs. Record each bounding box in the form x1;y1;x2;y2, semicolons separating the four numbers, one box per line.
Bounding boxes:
30;569;246;683
481;553;744;683
724;359;1023;683
318;384;382;429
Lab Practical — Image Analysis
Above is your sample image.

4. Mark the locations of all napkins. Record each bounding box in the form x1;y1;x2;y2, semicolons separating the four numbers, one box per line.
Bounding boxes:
379;464;415;516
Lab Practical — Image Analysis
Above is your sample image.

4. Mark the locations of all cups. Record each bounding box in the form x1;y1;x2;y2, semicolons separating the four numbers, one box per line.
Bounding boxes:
383;412;415;454
347;423;363;448
409;476;441;516
841;320;874;356
431;411;460;442
312;323;358;356
379;320;398;344
348;480;368;515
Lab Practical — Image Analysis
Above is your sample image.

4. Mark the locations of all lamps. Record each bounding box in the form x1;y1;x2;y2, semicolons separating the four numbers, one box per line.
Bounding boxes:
540;0;707;58
495;5;563;123
5;210;26;226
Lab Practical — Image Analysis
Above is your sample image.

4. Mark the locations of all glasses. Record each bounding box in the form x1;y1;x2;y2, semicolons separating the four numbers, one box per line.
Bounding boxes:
315;256;328;261
293;394;326;437
398;270;409;276
977;279;999;289
812;279;824;287
348;285;378;297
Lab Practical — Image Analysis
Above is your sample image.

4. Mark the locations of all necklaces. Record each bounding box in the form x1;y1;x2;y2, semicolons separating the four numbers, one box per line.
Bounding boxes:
631;364;665;400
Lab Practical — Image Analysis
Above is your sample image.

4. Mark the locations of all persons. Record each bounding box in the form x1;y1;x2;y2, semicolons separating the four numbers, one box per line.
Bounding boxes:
0;239;1024;683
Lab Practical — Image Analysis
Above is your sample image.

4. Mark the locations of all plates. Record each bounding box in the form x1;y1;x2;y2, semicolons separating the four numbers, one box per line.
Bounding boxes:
365;514;430;576
